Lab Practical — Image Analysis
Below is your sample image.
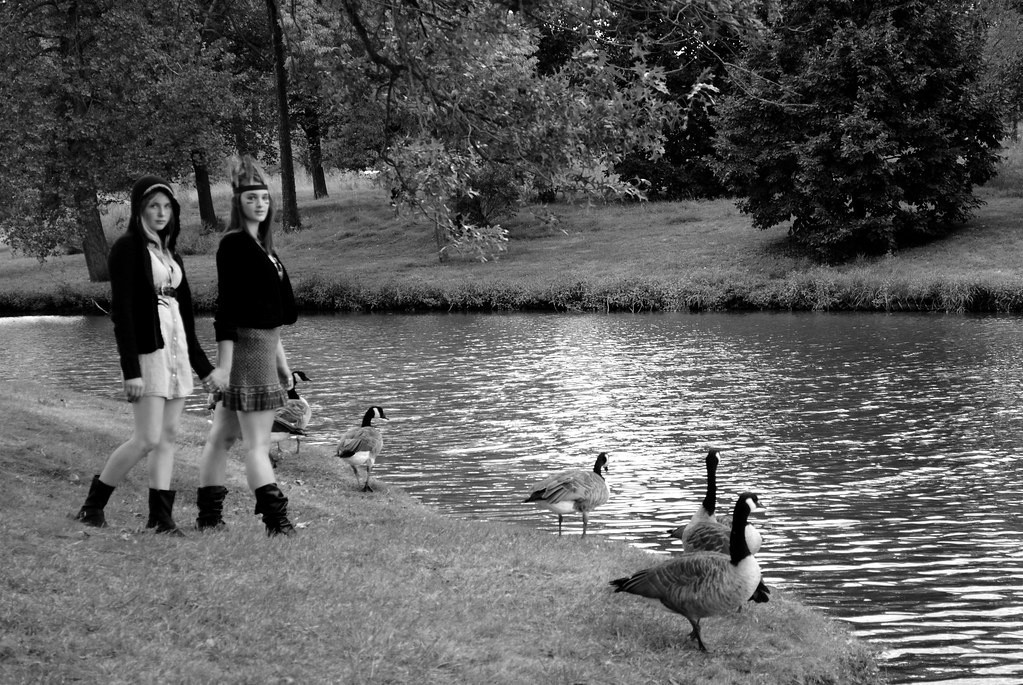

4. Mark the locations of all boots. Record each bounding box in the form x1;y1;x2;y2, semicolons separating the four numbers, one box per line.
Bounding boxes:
195;485;228;530
147;488;186;537
255;483;294;537
77;475;115;528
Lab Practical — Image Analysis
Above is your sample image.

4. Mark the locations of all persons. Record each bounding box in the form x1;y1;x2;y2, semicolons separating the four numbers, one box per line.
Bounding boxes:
198;169;299;540
75;176;226;539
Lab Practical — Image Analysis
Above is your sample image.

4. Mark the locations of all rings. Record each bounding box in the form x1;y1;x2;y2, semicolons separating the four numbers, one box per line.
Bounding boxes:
206;382;209;385
208;386;212;390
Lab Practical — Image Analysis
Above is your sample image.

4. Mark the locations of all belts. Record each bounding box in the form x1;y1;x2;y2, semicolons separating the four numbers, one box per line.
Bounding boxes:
157;286;175;297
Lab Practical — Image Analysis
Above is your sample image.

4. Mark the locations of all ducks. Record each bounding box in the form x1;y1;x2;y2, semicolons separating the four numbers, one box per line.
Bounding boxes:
607;448;771;656
517;452;609;539
332;406;390;493
207;371;312;469
